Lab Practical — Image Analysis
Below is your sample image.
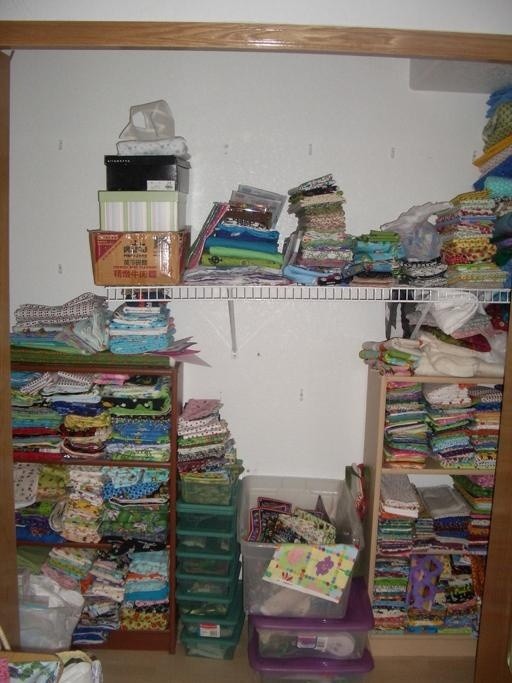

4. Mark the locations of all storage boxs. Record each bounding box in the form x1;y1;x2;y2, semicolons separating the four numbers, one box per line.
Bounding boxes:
177;529;237;556
177;573;237;598
243;635;376;682
88;156;193;286
176;550;235;577
175;595;236;619
237;471;364;622
178;629;240;662
177;493;237;533
253;575;375;665
178;473;231;504
180;615;241;639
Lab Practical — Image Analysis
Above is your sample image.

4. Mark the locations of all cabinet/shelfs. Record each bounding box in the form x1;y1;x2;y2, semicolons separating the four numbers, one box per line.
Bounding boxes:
11;342;176;653
362;366;505;642
